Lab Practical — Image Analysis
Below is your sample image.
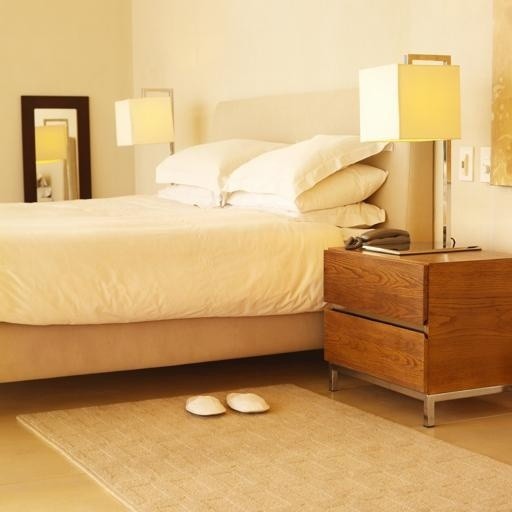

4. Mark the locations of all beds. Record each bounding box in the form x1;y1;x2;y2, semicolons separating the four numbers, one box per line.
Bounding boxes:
0;82;436;383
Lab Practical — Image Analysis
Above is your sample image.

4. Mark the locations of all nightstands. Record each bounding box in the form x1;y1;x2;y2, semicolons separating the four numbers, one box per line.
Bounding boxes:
322;241;512;426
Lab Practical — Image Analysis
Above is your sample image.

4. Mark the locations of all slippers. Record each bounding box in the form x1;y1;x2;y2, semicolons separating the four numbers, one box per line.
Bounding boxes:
185;395;227;417
226;392;271;415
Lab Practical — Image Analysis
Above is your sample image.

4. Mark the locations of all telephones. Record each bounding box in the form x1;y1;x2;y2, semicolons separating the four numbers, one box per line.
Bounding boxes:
357;228;410;250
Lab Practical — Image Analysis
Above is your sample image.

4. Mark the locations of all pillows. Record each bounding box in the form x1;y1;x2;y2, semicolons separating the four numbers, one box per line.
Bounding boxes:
219;133;394;195
154;183;222;206
227;163;390;212
273;201;389;228
154;137;295;192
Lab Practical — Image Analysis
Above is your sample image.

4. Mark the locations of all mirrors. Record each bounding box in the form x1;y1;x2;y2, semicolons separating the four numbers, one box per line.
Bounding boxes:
21;96;91;202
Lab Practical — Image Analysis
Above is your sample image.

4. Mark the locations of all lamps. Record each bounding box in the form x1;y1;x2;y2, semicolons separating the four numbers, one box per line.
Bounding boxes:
114;86;174;185
35;119;70;201
359;55;480;255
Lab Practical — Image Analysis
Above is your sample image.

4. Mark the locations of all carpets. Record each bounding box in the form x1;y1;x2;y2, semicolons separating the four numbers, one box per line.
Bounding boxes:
15;383;511;512
